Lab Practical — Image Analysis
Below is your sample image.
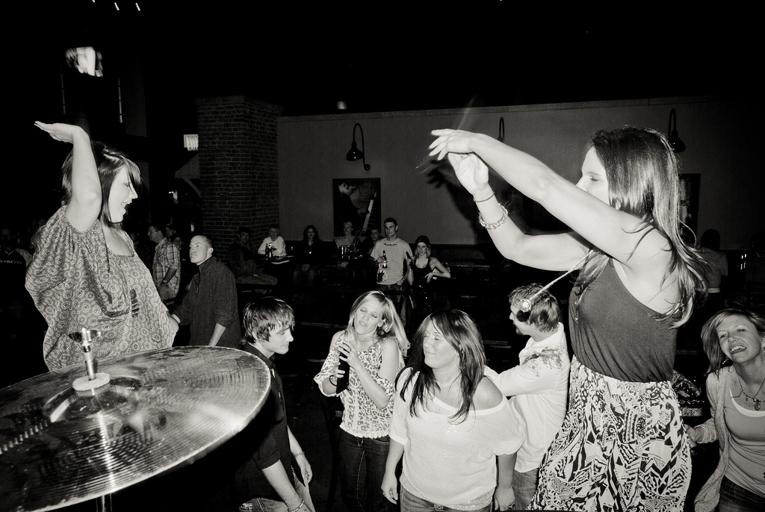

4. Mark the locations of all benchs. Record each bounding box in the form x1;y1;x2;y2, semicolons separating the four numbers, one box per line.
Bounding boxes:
276;239;549;337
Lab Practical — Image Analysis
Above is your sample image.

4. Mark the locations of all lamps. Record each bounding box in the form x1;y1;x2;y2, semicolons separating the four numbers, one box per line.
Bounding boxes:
666;106;686;154
346;123;370;171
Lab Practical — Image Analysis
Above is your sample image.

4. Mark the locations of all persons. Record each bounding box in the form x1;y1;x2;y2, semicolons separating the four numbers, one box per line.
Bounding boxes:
429;126;702;510
315;282;764;512
299;216;450;326
29;121;315;512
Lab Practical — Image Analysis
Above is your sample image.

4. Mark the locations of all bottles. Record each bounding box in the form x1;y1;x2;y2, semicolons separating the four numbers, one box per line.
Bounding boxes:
265;242;272;260
382;250;387;268
341;244;348;256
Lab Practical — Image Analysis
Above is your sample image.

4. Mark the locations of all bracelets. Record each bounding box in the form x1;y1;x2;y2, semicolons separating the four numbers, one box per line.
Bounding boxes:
473;193;496;203
479;203;507;230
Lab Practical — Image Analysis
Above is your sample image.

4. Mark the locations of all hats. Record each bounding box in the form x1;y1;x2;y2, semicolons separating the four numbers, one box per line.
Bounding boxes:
414;235;430;249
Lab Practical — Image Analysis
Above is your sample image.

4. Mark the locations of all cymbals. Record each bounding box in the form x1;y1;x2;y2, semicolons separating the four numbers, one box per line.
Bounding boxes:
0;344;270;512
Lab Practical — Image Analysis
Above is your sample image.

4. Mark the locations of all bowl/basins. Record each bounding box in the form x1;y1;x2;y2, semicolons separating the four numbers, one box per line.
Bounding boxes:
273;255;283;260
